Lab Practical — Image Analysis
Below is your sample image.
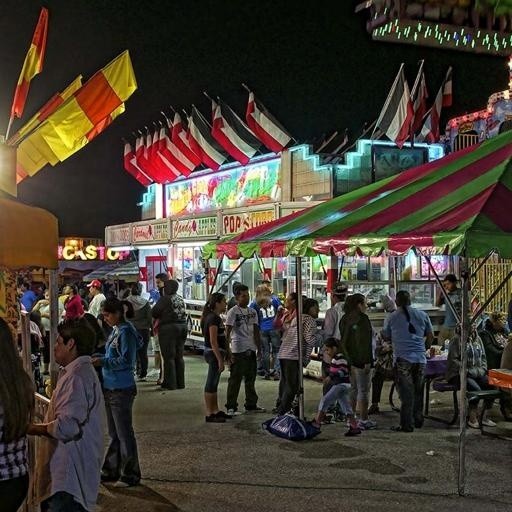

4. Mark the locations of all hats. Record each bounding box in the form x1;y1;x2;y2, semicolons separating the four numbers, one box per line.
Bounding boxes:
85;279;102;288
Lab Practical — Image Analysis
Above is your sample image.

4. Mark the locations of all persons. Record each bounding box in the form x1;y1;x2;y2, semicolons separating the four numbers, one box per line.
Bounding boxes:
382;289;434;433
272;281;375;436
1;318;36;510
367;331;395;415
389;284;396;301
436;275;512;429
25;316;105;511
20;272;189;390
91;297;143;490
201;280;284;423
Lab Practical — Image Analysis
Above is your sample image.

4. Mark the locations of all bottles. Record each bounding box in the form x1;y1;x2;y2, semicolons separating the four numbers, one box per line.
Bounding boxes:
445;338;451;354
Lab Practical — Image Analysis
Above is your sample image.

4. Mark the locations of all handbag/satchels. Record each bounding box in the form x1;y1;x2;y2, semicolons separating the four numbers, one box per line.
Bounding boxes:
272;311;283;328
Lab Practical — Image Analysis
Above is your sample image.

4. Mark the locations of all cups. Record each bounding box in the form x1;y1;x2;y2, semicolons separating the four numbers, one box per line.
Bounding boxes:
429;347;436;359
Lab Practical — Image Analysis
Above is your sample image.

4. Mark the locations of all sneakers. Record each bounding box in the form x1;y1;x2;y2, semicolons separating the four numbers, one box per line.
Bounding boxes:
99;467;139;489
200;402;497;435
134;370;186;391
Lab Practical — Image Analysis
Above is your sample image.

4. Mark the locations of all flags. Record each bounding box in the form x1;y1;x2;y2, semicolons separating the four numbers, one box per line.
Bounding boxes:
124;92;293;187
13;8;137;189
409;70;429;150
374;66;415;151
419;71;453;146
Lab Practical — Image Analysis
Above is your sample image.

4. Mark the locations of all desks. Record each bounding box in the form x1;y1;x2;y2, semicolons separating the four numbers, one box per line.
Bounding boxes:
389;347;448;423
488;368;512;390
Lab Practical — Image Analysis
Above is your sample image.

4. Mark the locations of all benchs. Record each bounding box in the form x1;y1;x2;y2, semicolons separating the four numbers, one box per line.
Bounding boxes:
468;390;501;433
432;380;459;424
